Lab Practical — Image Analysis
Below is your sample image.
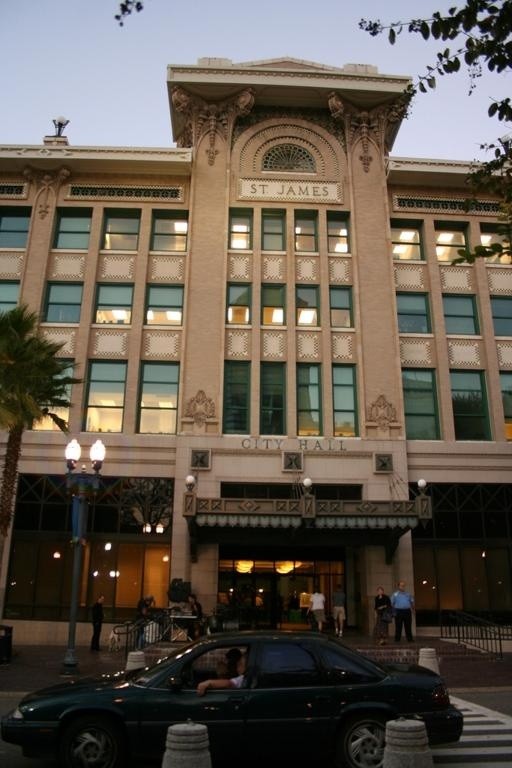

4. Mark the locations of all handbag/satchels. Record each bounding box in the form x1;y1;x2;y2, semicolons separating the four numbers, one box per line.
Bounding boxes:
380;608;393;624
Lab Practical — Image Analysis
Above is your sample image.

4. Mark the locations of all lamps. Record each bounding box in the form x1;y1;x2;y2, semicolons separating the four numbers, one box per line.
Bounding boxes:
52;116;70;136
418;478;428;495
301;475;314;495
184;473;197;494
233;560;301;576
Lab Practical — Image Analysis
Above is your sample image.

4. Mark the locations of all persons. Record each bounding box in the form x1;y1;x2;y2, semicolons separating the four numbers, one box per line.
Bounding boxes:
299;587;309;623
187;596;202;640
307;585;326;632
332;584;346;637
91;594;105;651
195;653;248;697
374;586;391;646
135;595;154;646
390;579;417;642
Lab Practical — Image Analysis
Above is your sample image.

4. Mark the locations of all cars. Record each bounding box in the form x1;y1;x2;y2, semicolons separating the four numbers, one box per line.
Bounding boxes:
0;630;463;767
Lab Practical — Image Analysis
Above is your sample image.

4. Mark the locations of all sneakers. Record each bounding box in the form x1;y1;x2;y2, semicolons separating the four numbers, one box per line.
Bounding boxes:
381;638;415;644
334;630;344;637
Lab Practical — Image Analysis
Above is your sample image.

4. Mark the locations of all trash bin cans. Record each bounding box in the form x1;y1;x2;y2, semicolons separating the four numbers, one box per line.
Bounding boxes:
0;625;13;665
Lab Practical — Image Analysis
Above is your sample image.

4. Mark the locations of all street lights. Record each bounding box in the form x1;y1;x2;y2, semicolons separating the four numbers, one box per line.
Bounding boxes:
61;437;106;676
